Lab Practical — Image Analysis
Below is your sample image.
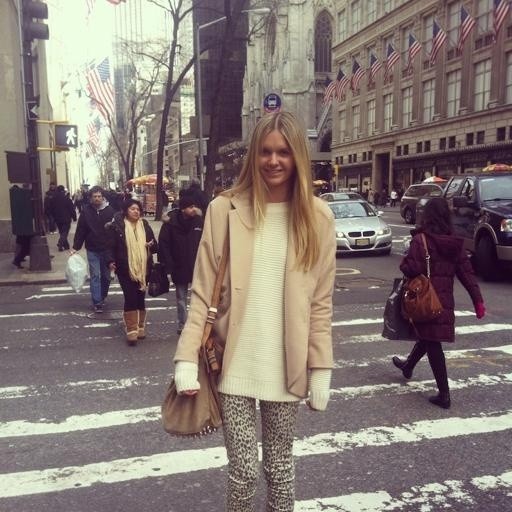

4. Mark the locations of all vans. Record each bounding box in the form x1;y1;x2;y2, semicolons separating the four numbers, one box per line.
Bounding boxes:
414;169;512;282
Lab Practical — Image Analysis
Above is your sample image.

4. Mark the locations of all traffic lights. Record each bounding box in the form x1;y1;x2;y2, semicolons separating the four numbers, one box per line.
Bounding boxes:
52;124;79;149
23;2;50;43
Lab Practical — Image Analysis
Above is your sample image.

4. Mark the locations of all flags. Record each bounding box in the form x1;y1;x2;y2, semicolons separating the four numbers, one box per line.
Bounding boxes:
369;53;382;87
384;44;399;80
430;19;448;64
323;75;336;105
492;0;509;43
408;33;422;71
86;0;96;20
82;57;116;155
351;60;365;95
335;70;350;104
458;6;476;51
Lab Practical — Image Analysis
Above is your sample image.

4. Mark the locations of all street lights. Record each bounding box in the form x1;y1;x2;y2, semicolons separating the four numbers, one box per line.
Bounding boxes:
194;6;273;195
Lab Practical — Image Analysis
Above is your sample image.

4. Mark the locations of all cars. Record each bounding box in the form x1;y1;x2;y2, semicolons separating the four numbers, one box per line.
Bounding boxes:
319;188;377;212
399;181;448;224
326;198;393;257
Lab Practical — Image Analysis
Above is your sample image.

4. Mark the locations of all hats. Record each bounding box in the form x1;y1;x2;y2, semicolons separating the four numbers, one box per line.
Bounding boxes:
180;196;196;209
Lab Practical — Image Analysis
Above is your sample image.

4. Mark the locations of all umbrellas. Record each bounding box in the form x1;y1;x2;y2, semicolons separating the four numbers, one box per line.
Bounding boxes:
423;176;448;191
482;162;512;174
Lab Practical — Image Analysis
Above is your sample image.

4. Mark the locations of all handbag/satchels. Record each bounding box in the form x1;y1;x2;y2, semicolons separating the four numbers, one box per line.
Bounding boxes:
68;253;87;292
147;262;169;298
382;274;443;341
161;338;223;437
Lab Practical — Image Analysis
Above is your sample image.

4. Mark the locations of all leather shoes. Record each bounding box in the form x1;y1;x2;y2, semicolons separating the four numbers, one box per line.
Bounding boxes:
393;356;412;379
13;259;26;267
429;396;450;408
57;243;69;251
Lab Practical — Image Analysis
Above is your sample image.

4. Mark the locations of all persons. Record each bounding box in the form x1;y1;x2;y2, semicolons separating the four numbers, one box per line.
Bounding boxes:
173;113;333;510
11;183;211;344
360;188;399;208
394;198;485;408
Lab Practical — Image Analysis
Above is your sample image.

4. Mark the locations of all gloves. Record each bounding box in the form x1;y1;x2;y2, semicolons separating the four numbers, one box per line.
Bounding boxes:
476;304;486;319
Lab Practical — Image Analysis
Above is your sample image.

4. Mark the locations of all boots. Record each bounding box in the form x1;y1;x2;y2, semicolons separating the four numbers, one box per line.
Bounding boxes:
124;309;146;341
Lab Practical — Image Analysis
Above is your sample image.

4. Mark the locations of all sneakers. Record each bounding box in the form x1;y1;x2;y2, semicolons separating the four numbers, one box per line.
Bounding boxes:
95;303;104;313
